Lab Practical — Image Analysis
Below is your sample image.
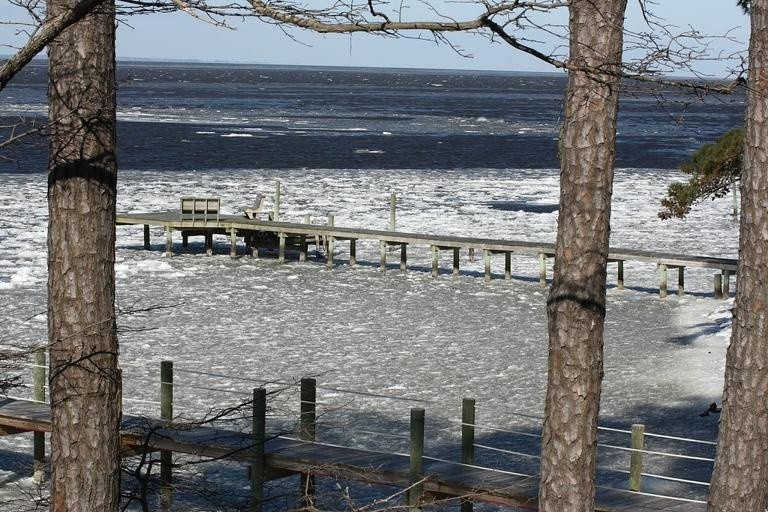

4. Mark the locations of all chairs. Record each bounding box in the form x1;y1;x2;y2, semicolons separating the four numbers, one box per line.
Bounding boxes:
241;194;266;220
